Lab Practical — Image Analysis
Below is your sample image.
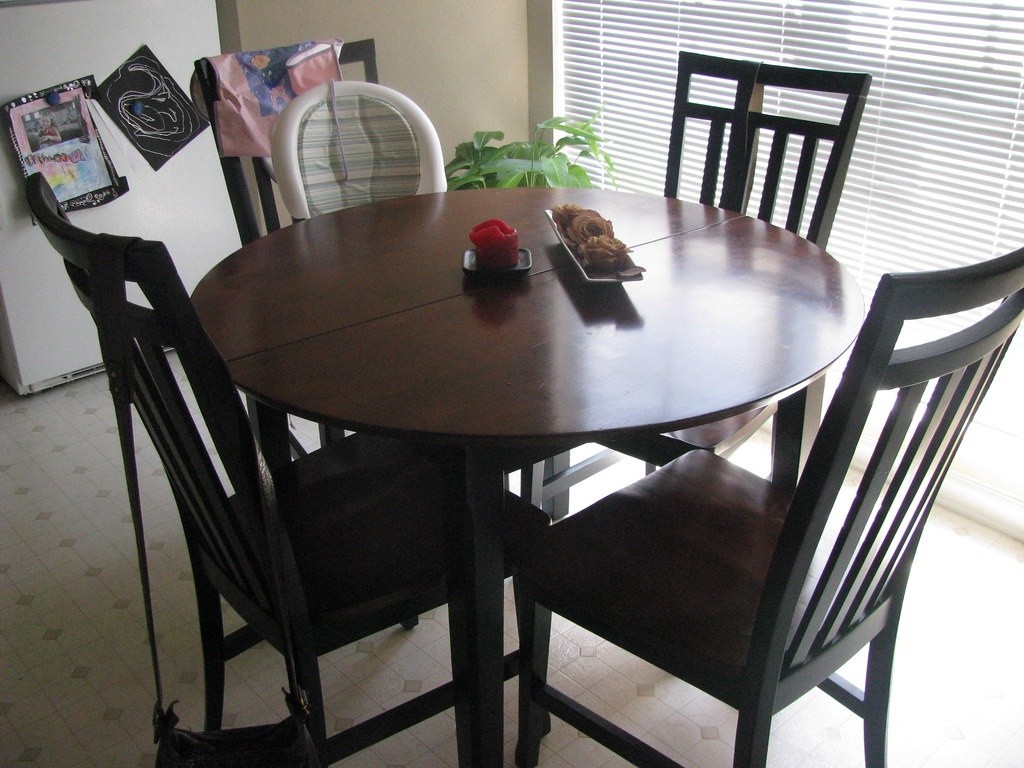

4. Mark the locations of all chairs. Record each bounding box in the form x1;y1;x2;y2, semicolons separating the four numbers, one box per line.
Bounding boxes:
190;32;384;466
521;44;874;508
271;81;448;219
509;250;1024;768
22;168;557;768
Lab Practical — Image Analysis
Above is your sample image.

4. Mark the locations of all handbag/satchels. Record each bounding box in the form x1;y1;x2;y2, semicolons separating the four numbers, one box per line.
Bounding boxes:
154;689;320;768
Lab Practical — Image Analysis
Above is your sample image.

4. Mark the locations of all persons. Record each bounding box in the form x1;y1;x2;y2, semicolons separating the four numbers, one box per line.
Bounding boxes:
38;110;62;150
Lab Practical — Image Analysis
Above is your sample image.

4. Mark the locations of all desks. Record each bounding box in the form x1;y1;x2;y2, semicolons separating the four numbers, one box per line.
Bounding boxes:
187;180;869;768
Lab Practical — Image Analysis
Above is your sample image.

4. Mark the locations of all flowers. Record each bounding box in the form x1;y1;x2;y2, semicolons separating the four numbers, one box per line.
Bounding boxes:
552;194;629;266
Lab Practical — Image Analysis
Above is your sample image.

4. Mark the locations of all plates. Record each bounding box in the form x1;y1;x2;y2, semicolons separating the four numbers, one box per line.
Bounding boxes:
544;209;643;282
463;247;532;271
